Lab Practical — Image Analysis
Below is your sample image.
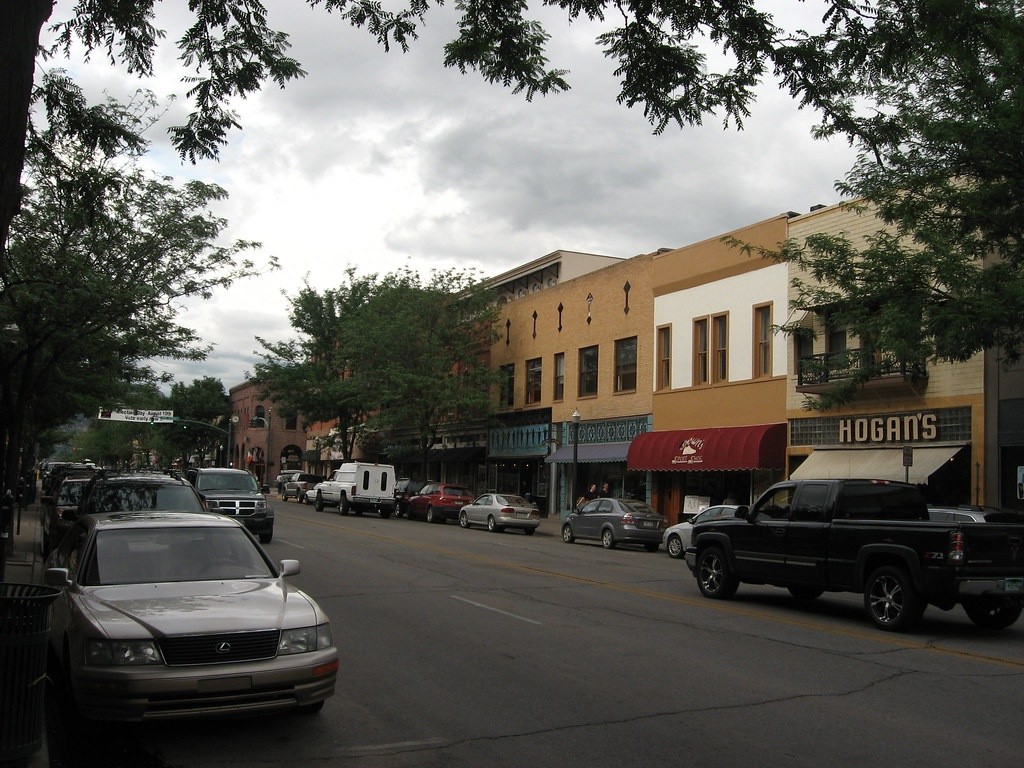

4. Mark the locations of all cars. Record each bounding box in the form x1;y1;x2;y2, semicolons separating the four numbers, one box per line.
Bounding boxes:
42;462;130;551
662;504;765;559
459;493;541;533
561;497;668;549
45;507;340;733
304;481;322;506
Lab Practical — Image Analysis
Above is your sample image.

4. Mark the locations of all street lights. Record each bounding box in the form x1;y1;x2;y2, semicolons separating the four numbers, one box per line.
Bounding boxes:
571;408;582;514
253;408;273;495
315;436;320;462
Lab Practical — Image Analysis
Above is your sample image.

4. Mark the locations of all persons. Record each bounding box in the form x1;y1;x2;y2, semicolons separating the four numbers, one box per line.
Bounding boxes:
277;474;283;494
723;492;738;505
576;484;597;507
598;483;610;498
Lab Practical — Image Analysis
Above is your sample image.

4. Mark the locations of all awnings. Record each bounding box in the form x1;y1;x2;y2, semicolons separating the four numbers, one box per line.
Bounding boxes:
544;441;633;463
789;447;964;485
627;422;788;471
386;447;485;465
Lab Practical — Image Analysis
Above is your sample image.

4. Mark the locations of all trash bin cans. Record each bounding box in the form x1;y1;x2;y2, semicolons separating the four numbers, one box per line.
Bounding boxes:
0;582;65;763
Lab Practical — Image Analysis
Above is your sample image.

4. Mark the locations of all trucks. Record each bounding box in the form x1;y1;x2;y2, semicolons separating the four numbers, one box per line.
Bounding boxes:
314;462;396;515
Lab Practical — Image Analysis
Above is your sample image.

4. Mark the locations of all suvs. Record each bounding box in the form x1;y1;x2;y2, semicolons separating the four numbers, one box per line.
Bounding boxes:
62;470;229;528
186;466;275;545
405;481;476;523
377;477;432;517
928;504;1023;525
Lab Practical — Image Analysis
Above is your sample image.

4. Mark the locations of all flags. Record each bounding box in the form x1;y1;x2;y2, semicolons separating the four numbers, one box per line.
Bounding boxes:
247;446;264;464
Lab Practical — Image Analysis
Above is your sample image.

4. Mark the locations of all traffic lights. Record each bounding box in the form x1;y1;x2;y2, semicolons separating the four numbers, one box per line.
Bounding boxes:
151;417;154;425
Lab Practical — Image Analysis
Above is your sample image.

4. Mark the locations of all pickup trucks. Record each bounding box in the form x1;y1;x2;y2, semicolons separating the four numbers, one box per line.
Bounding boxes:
683;478;1024;633
275;470;325;503
